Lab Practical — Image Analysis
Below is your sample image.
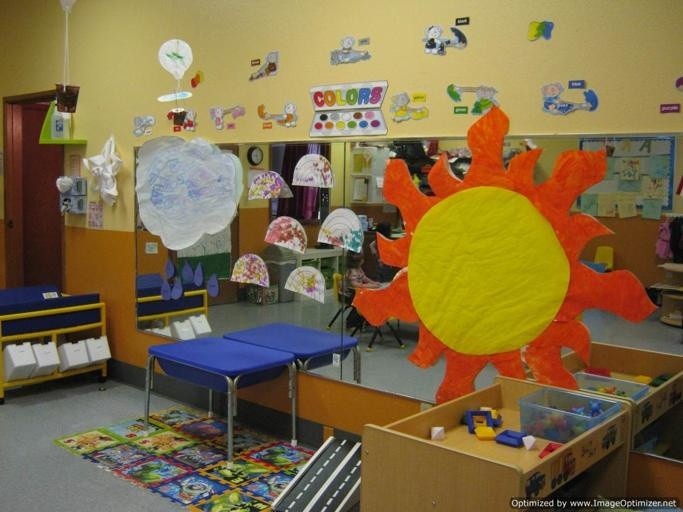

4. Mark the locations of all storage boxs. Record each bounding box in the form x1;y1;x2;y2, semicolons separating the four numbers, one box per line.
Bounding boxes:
145;314;212;341
3;336;112;379
519;386;622;446
573;372;649;402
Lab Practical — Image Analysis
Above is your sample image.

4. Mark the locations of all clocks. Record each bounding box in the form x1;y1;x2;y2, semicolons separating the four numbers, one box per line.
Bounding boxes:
247;147;263;166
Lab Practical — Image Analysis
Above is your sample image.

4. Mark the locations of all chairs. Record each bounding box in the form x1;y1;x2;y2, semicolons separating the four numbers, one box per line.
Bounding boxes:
351;310;406;351
326;273;352;330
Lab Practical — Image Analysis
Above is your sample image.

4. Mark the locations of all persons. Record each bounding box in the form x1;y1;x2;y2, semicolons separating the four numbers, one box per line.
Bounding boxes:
338;247;382;326
368;219;400;276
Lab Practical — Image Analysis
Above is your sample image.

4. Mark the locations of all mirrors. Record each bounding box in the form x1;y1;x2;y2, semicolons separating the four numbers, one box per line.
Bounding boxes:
134;132;683;464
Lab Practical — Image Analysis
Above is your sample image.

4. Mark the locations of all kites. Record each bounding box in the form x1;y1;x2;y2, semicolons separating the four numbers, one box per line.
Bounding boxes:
460;366;683;450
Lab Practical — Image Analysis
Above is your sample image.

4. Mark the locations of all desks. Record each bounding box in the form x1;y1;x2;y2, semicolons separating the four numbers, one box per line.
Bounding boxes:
223;322;362;386
361;376;632;512
145;337;298;470
525;341;683;454
